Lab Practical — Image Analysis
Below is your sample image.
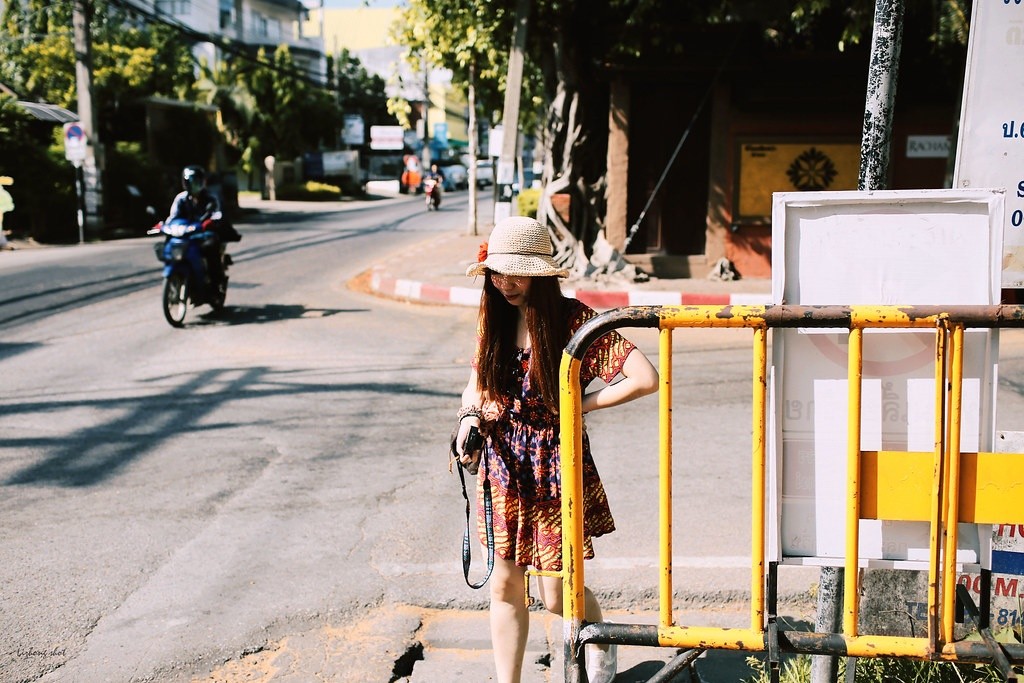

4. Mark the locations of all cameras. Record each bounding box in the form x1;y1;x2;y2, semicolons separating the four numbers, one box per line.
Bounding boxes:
465;426;485;457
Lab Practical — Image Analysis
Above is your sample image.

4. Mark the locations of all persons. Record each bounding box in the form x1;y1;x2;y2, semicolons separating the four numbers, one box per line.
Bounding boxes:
152;166;223;296
453;215;663;683
424;165;444;201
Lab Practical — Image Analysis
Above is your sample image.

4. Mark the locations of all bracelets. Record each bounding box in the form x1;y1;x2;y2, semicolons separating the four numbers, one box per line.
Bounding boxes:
459;413;482;422
456;404;482;417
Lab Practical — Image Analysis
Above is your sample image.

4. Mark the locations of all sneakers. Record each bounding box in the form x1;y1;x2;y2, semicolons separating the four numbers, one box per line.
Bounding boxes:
587;620;617;683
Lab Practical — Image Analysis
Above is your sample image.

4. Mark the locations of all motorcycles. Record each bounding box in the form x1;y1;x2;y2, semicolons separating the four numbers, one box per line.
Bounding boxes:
425;178;441;211
143;201;244;327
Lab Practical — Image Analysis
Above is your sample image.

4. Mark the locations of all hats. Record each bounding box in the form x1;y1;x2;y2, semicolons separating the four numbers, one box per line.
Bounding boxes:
465;218;568;280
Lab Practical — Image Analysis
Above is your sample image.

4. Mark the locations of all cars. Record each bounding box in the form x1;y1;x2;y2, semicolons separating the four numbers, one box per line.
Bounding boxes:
435;155;538;202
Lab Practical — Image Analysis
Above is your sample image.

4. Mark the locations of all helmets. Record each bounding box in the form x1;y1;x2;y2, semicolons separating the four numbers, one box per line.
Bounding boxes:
183;166;206;192
